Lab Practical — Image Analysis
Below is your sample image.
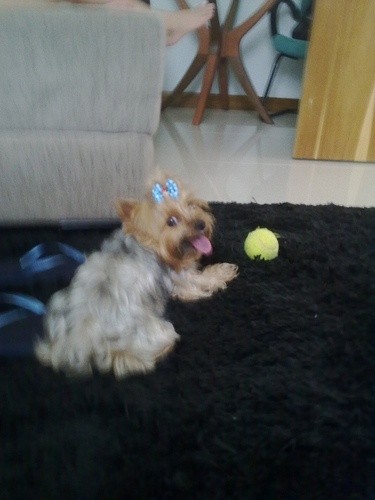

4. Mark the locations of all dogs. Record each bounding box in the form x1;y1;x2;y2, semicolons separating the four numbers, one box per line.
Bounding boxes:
29;173;241;379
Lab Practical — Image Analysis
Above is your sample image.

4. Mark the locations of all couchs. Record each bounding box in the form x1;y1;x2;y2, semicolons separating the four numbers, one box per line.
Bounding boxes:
0;0;168;227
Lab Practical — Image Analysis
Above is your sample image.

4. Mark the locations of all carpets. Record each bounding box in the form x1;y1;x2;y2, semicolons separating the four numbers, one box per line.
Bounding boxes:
1;201;374;500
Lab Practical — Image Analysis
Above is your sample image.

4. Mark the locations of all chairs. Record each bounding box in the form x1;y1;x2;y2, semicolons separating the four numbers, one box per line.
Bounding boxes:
256;0;314;110
